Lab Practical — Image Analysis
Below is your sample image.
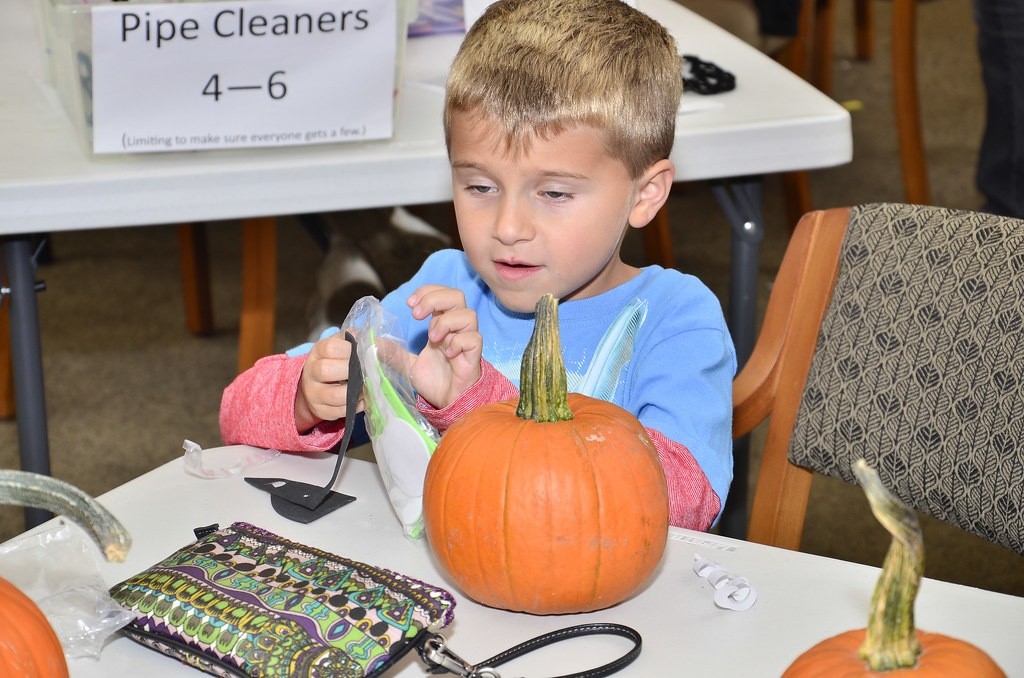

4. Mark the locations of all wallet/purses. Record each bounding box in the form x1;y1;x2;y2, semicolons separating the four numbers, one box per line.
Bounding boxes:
105;519;456;678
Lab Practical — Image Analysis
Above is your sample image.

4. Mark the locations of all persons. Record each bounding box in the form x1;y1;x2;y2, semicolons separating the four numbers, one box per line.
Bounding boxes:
217;3;737;534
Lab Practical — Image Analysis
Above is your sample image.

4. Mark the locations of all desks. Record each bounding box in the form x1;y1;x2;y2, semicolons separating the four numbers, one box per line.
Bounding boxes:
0;440;1023;677
0;3;851;526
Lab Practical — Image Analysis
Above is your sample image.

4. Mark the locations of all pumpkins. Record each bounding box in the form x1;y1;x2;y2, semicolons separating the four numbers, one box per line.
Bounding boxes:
422;293;669;616
780;458;1007;678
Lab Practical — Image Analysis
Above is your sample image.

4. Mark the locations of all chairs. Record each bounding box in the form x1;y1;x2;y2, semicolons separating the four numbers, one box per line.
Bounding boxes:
728;202;1023;597
